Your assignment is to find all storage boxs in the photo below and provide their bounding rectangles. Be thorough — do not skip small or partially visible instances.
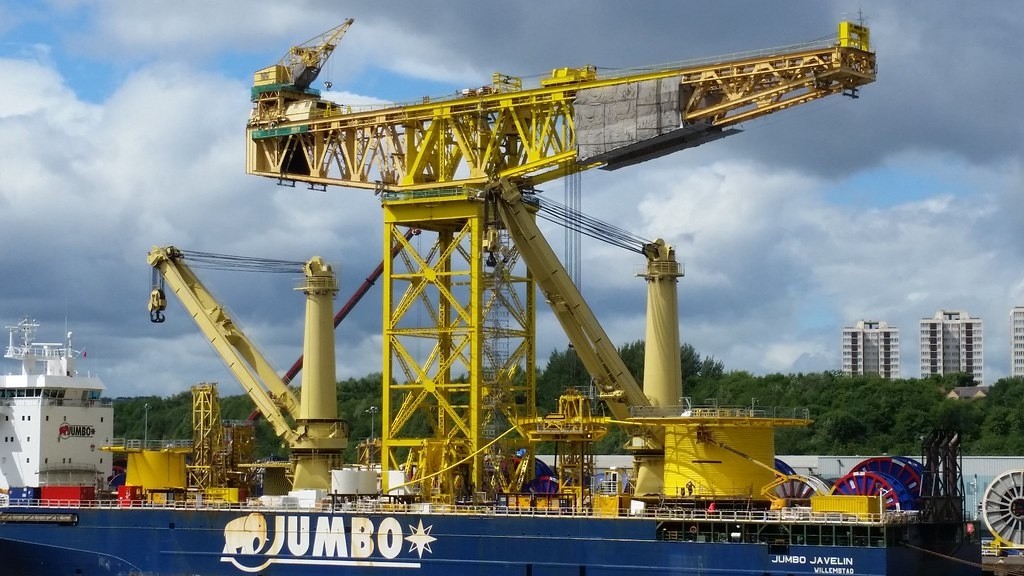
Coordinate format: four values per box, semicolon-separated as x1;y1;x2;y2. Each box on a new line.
40;486;94;508
811;495;886;521
498;495;569;515
9;487;40;506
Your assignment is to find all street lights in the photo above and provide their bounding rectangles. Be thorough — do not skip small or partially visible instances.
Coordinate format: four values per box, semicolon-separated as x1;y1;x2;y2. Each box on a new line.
144;401;152;453
364;405;378;465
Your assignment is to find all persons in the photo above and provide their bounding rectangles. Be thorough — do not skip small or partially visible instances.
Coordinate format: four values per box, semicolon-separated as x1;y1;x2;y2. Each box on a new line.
686;480;695;495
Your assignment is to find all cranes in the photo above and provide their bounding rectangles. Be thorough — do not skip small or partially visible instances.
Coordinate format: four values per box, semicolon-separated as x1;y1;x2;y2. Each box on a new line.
242;13;880;504
145;243;350;498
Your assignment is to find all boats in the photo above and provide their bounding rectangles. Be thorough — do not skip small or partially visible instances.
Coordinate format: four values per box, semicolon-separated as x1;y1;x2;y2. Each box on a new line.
0;14;985;576
